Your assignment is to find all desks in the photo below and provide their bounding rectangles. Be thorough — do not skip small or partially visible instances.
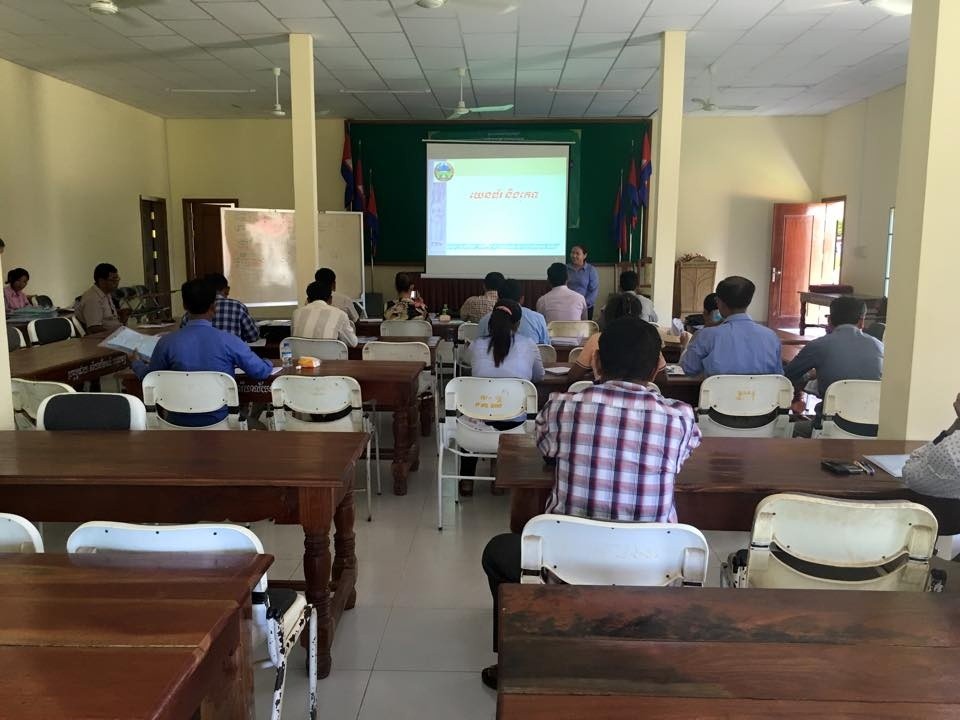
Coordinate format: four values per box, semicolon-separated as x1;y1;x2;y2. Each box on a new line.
114;359;426;496
252;337;440;431
0;431;370;676
770;329;816;364
0;551;274;720
530;362;703;407
496;584;960;719
555;336;682;363
9;318;183;392
0;285;960;720
254;317;463;376
493;434;928;532
5;307;74;347
797;291;888;335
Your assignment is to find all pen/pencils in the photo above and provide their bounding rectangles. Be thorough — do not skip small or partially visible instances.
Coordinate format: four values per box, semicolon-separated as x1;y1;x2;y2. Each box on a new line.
856;462;869;472
431;319;440;320
860;462;873;472
864;462;875;472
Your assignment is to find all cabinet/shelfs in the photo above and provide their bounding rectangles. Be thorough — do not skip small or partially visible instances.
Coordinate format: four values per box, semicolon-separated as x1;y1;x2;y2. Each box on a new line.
674;255;717;318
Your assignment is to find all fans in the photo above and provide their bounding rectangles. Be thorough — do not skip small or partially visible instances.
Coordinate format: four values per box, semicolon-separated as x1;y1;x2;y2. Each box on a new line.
432;68;514;120
820;0;912;18
687;63;759;112
377;0;518;15
69;0;166;27
262;67;288;117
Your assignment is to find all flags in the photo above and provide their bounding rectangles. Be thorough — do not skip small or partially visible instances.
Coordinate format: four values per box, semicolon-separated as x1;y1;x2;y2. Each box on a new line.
611;132;652;255
340;131;378;257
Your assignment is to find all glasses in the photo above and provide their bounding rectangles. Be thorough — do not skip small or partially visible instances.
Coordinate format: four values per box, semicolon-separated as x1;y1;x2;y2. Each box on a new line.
104;277;120;284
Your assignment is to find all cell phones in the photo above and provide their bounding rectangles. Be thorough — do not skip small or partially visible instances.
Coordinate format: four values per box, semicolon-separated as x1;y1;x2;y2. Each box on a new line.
840;463;864;474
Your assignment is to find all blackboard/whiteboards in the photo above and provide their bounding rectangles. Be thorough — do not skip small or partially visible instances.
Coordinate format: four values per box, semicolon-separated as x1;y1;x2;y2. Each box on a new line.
220;207;364;307
344;119;652;266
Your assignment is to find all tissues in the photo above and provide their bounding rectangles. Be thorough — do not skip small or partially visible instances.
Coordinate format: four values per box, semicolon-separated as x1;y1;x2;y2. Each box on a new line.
297;357;321;368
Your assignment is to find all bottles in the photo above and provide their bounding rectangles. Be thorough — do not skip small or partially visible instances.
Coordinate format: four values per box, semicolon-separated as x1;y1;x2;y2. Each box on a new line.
281;343;293;367
442;304;449;314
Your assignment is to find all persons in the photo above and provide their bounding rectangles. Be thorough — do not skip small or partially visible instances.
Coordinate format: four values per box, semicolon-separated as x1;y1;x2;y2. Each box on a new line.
479;279;551;346
180;273;259;342
459;299;544;496
481;315;702;689
783;297;884;439
679;275;784;429
76;263;124;334
565;244;599;321
290;281;358;348
305;268;360;328
567;293;668;388
620;271;658;321
127;279;272;427
536;262;588;323
460;271;505;324
902;393;960;594
383;272;432;324
3;268;32;311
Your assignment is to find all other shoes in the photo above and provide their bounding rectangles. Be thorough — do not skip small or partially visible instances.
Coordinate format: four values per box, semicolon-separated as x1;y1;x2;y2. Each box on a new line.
482;664;500;690
492;483;504;496
458;479;473;497
